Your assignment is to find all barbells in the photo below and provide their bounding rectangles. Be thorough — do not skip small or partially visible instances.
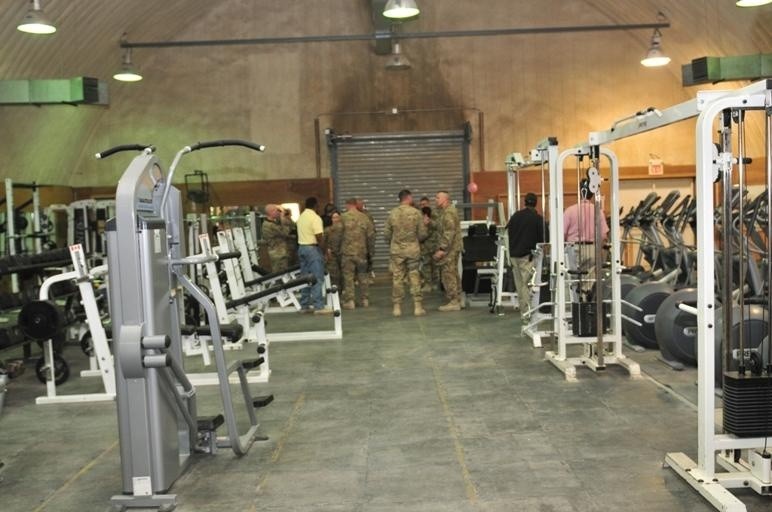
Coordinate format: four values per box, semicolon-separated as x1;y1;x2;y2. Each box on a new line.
18;290;104;342
35;330;96;386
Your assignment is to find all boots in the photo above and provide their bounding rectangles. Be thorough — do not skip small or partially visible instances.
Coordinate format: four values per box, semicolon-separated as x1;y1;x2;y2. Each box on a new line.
413;301;426;316
343;300;355;309
361;299;369;306
393;304;401;316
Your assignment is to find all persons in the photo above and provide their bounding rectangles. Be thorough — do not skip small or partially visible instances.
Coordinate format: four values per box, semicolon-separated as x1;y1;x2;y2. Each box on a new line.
258;197;377;314
384;189;426;317
432;191;461;312
562;178;609;304
504;192;550;324
420;197;439;293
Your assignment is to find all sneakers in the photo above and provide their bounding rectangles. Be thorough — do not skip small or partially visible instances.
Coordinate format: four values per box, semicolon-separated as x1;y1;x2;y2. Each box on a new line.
438;295;466;311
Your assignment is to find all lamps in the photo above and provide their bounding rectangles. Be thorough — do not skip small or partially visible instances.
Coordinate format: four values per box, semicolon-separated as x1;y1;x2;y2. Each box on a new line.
111;44;146;86
17;1;60;35
383;40;412;72
380;0;423;21
639;26;674;68
